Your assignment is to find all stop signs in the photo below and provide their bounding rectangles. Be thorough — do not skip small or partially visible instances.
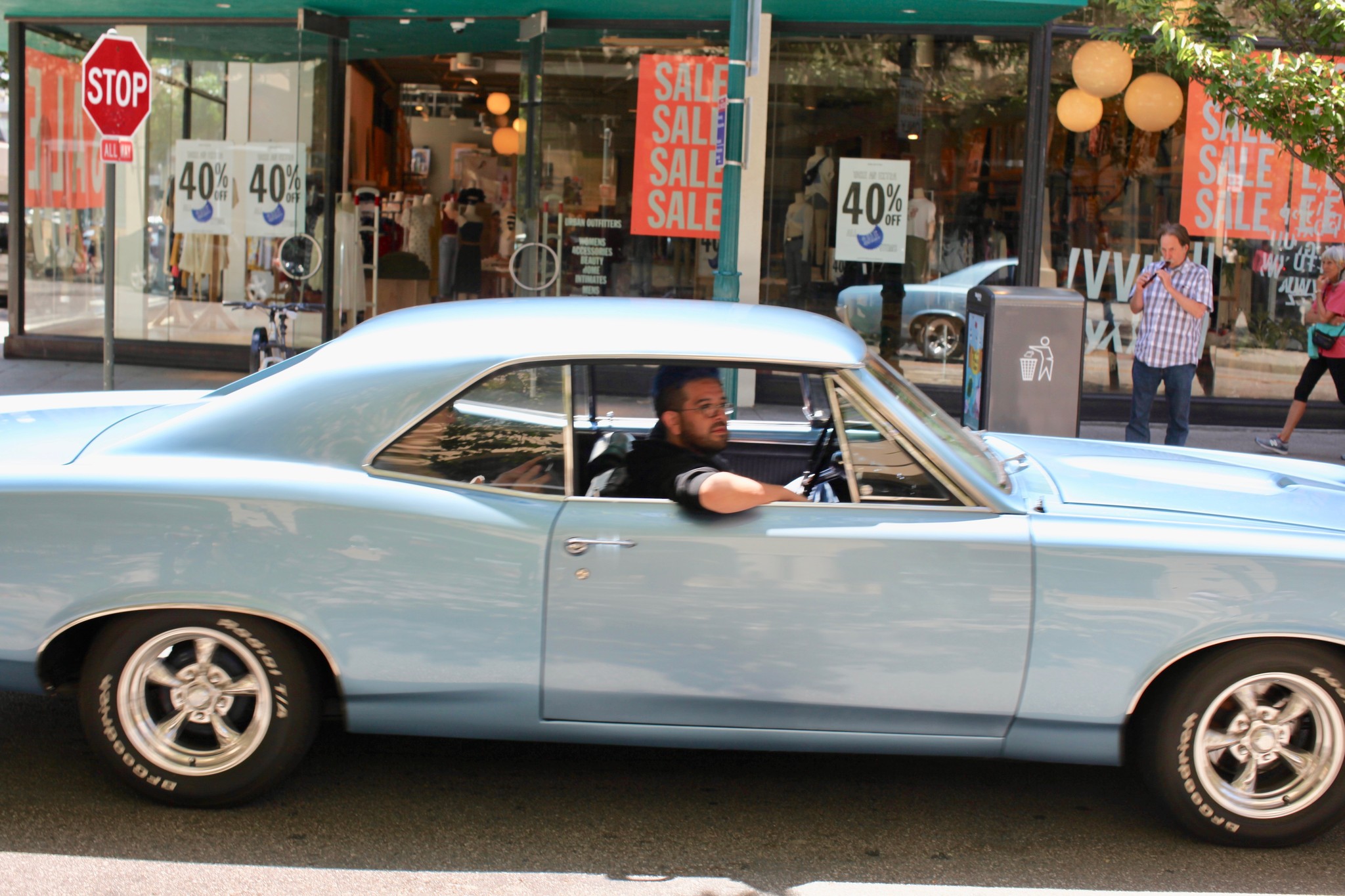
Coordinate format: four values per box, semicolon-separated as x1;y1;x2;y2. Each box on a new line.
80;36;152;137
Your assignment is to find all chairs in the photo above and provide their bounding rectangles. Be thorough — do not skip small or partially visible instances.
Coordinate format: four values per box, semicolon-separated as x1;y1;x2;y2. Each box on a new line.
590;428;639;501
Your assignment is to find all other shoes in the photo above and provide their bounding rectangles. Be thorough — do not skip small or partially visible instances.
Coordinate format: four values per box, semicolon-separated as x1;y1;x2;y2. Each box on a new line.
1255;434;1289;455
1341;454;1345;460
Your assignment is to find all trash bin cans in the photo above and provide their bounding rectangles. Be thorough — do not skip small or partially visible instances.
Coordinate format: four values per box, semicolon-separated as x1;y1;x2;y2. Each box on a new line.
960;283;1088;438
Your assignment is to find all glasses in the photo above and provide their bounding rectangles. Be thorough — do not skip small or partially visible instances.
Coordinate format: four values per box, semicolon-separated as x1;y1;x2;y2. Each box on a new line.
681;403;734;418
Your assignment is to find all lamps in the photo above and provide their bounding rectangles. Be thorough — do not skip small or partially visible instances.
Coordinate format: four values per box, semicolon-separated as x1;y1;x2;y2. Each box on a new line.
915;34;1183;134
415;53;527;157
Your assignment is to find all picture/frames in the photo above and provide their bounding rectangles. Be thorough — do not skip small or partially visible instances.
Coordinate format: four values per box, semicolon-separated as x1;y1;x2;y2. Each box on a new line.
450;143;478;180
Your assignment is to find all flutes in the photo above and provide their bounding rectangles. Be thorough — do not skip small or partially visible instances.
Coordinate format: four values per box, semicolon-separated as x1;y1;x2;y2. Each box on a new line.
1141;261;1171;289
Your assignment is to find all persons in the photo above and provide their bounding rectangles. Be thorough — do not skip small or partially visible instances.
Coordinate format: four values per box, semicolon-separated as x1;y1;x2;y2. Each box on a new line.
1126;222;1214;447
1251;240;1279;311
370;401;552;494
619;365;814;514
1257;247;1344;459
1222;239;1238;289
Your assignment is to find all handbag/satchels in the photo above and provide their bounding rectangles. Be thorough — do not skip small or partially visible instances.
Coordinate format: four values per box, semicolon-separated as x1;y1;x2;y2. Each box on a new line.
804;165;818;186
1312;329;1336;350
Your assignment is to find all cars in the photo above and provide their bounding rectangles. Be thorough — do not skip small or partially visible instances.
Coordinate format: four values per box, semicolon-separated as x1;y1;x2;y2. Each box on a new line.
835;256;1023;365
0;294;1344;851
